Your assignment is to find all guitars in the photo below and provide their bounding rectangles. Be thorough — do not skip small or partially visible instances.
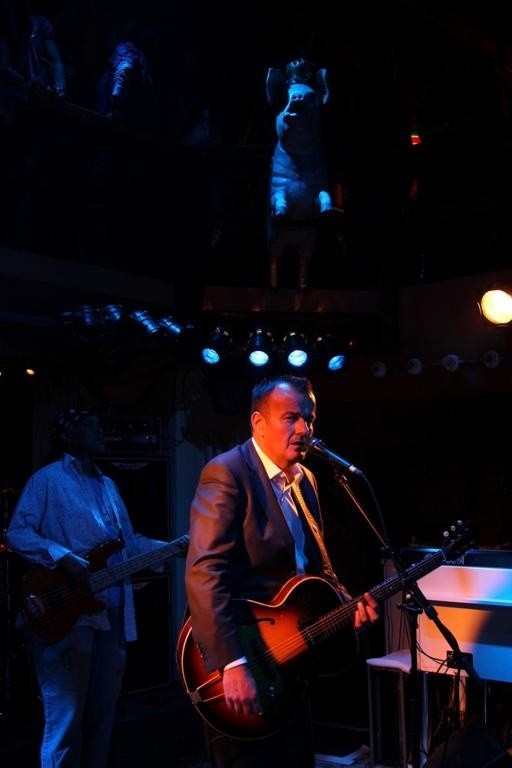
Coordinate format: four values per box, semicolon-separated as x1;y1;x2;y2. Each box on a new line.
175;519;477;741
2;535;189;652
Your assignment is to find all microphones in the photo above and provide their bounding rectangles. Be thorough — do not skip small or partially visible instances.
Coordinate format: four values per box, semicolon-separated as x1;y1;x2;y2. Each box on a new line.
305;437;363;477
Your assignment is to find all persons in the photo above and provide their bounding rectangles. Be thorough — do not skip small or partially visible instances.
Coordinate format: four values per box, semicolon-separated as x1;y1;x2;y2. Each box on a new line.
5;410;190;768
183;374;380;767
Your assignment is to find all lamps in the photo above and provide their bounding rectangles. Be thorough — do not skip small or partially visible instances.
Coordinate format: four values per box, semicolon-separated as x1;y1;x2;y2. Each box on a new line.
198;326;350;375
